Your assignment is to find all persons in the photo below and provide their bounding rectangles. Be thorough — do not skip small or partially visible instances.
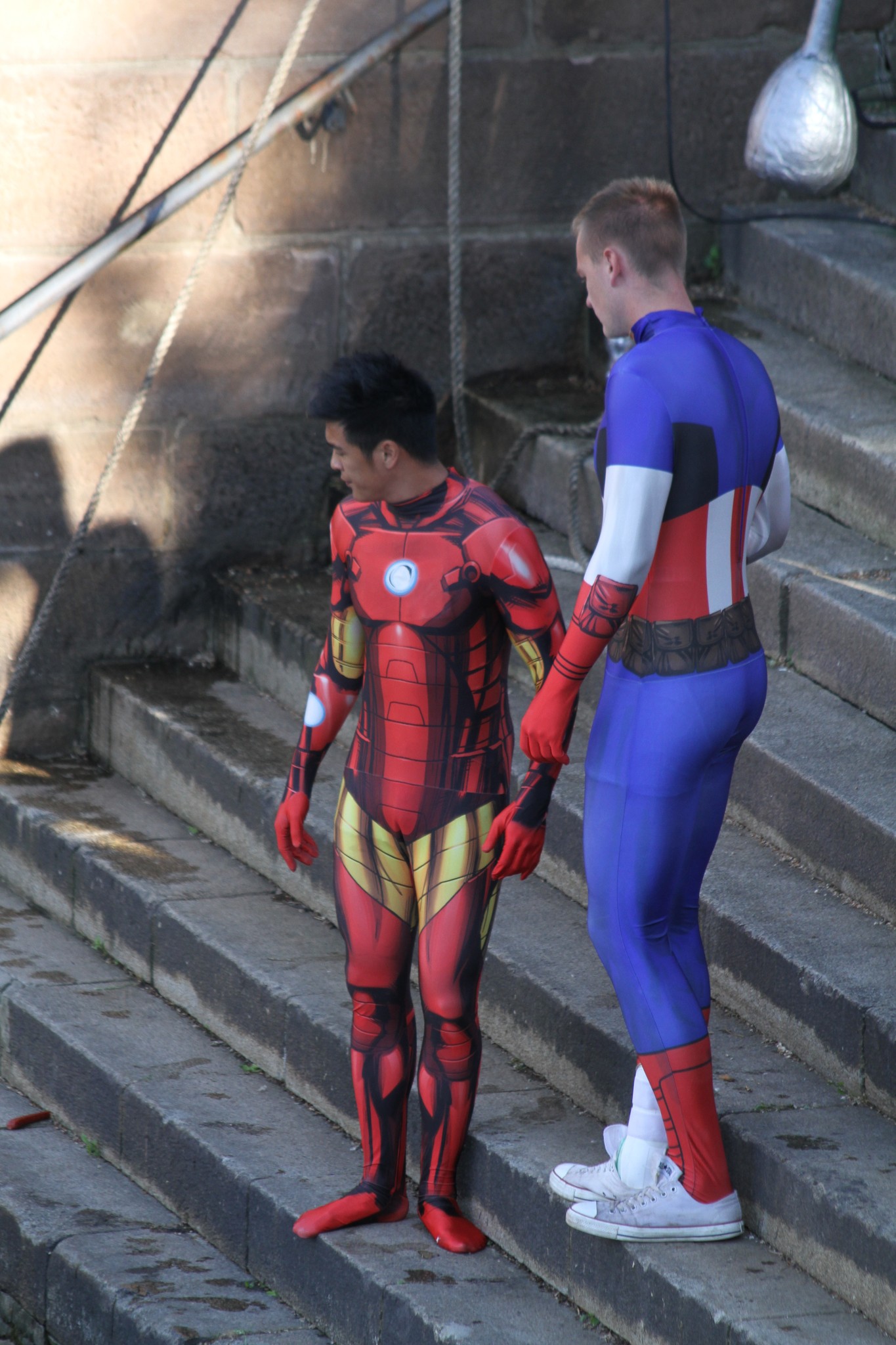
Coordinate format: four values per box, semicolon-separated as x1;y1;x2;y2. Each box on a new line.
518;178;794;1241
273;353;577;1259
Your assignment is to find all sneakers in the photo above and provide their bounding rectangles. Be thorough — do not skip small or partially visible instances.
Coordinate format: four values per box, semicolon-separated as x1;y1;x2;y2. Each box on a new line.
549;1123;646;1204
566;1154;745;1243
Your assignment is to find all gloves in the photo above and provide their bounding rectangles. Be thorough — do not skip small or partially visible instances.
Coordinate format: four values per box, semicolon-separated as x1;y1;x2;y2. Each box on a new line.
274;790;320;873
519;681;580;765
481;795;548;881
